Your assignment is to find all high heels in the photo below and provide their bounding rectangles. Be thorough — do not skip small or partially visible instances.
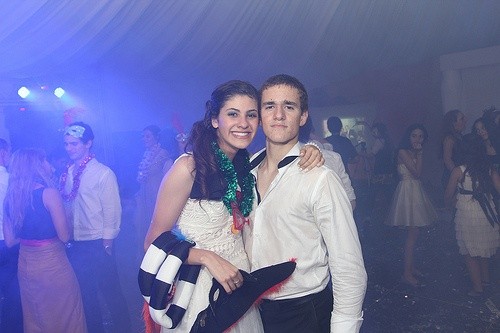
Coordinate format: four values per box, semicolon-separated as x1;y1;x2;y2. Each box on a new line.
400;266;426;287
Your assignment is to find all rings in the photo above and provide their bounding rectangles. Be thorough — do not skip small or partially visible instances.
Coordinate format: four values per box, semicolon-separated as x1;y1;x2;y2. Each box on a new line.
234;279;241;284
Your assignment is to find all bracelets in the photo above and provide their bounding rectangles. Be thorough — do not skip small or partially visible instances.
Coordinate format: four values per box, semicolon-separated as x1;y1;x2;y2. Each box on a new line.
103;244;112;249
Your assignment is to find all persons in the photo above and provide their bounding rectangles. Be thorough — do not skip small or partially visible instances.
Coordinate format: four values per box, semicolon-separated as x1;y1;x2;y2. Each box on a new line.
0;138;23;333
397;124;438;288
2;147;88;333
491;111;500;126
135;125;172;270
58;122;132;333
324;116;360;179
472;119;500;175
55;130;69;188
144;80;325;333
358;123;394;220
442;110;467;239
444;134;500;298
241;74;367;333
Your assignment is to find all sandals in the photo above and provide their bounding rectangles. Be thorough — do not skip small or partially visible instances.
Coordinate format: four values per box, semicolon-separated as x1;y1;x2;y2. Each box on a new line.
463;281;491;297
